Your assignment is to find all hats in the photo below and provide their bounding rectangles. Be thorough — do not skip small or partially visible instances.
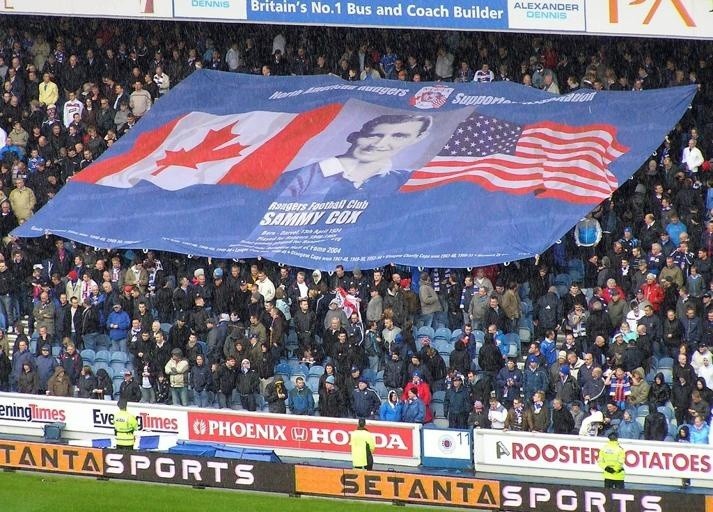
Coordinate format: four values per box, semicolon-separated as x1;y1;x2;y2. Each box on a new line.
0;24;713;440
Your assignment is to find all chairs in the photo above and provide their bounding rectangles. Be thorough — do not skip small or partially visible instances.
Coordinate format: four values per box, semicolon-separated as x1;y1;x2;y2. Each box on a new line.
29;257;672;445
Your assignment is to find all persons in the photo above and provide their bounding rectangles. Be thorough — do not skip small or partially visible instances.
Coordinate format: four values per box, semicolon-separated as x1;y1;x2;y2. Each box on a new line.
276;115;433;197
1;74;161;399
552;84;712;274
597;432;625;488
114;399;137;450
0;15;712;89
145;253;712;446
351;418;374;470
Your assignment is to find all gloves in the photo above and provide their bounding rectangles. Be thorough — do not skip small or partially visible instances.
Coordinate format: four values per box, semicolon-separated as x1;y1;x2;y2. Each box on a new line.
605;467;615;473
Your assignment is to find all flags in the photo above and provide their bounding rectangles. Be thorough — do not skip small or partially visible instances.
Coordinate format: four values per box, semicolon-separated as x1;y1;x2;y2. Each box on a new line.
72;103;342;191
399;110;629;203
